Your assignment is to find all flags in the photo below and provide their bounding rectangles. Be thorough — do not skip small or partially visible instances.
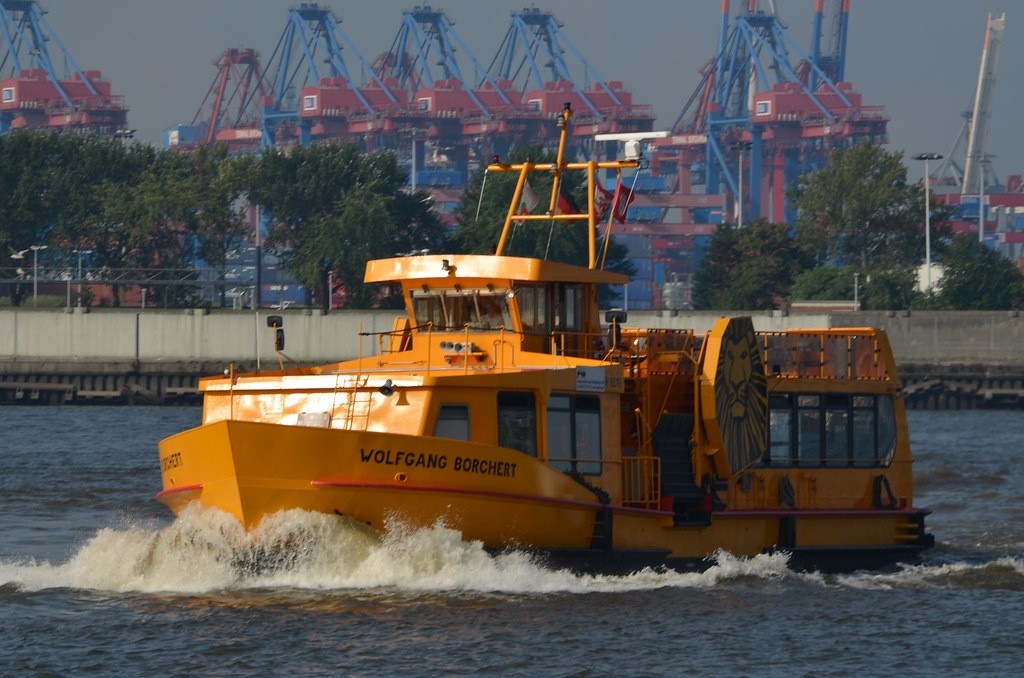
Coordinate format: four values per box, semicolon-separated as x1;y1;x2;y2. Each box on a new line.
515;179;539;227
594;180;613;226
556;182;583;225
613;184;634;224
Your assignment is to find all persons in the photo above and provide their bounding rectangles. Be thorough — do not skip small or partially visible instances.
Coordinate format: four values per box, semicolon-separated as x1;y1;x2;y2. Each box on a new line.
10;274;23;307
479;303;505;329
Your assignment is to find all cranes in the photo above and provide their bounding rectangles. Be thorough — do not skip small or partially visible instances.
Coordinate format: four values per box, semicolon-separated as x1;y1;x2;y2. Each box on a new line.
0;1;893;311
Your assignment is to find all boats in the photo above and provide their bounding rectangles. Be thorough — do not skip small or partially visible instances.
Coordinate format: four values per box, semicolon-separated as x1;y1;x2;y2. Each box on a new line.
154;100;934;580
